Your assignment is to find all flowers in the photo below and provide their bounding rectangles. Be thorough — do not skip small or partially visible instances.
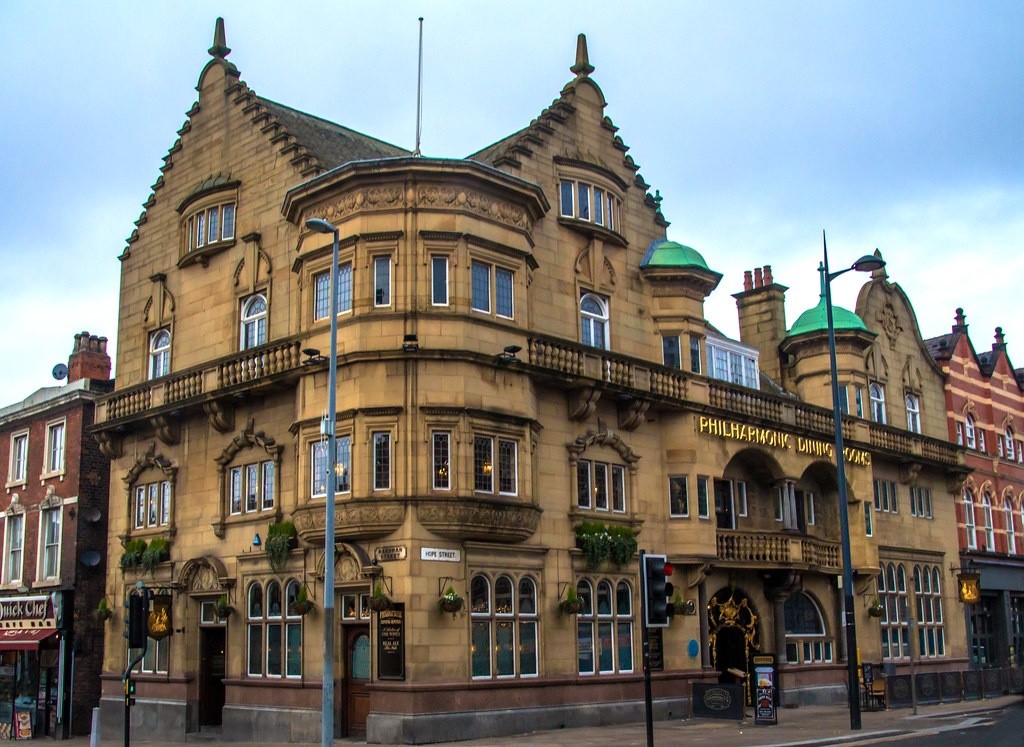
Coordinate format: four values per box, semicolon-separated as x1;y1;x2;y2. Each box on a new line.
872;600;883;611
438;586;468;620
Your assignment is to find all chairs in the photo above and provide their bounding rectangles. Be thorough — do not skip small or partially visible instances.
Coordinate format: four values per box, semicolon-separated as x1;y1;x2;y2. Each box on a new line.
844;675;886;711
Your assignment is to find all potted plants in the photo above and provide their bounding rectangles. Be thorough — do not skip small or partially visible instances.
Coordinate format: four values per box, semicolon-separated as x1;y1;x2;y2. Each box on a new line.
558;580;585;615
672;589;689;615
368;579;390;612
90;598;111;618
290;584;315;615
212;595;236;617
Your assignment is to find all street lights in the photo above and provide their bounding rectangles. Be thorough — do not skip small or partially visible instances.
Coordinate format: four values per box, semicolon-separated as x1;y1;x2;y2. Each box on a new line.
303;217;336;745
817;228;887;730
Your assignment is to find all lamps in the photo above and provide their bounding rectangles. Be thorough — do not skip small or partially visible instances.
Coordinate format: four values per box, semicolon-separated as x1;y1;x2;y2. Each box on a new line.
303;349;320;356
503;345;522;353
403;334;419;342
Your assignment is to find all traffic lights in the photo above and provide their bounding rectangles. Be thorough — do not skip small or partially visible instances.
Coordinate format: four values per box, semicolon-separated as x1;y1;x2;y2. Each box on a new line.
640;553;674;630
123;592;142;650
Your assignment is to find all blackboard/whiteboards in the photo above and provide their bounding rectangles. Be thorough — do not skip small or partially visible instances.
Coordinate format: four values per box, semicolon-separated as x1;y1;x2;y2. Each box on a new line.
755;687;774;719
